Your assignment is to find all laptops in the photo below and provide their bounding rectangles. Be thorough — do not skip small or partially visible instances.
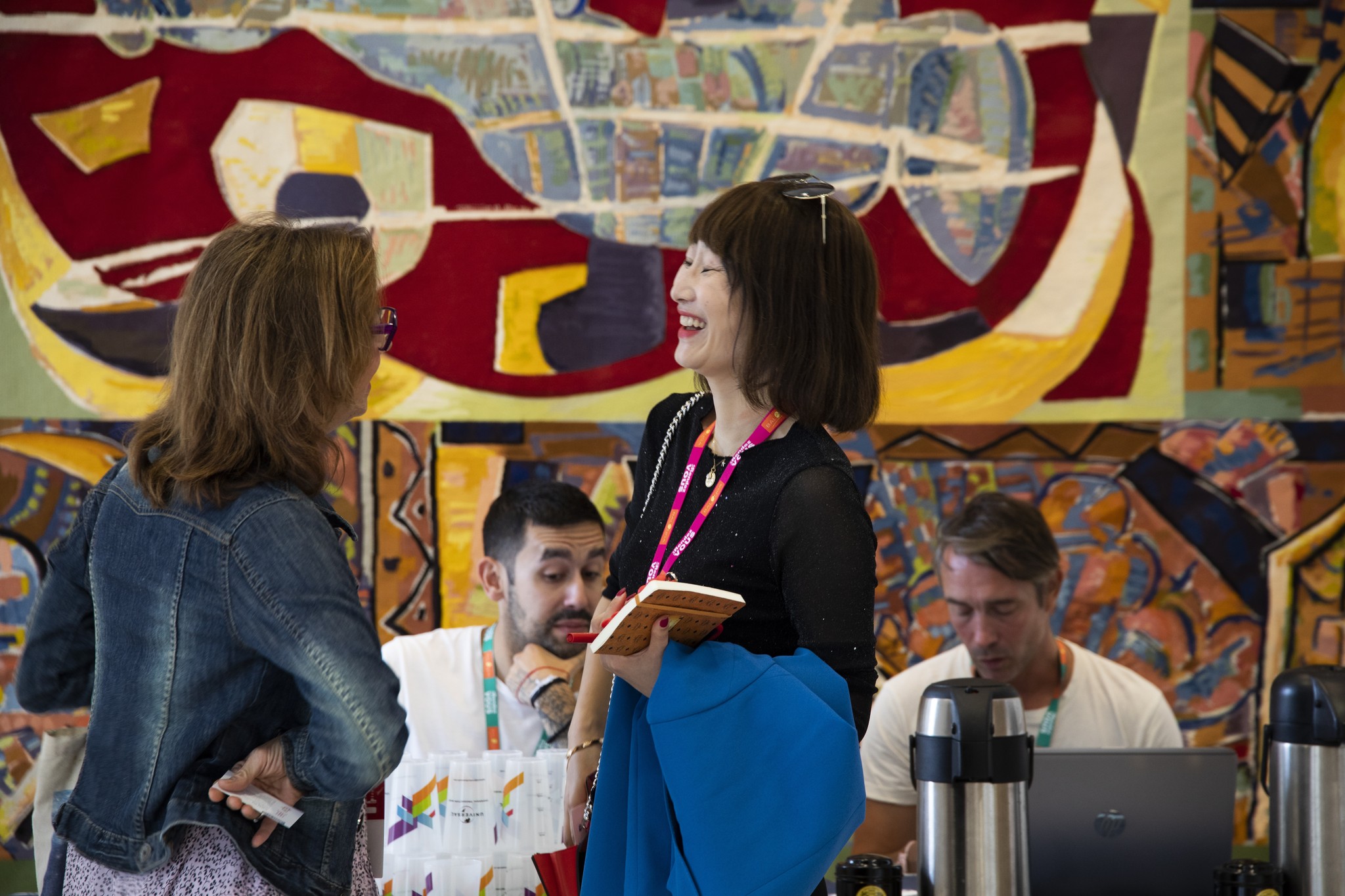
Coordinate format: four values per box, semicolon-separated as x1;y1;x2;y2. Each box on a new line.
1027;747;1237;896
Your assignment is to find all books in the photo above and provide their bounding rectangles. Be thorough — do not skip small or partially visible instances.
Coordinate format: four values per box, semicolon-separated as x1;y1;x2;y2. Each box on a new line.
589;580;746;655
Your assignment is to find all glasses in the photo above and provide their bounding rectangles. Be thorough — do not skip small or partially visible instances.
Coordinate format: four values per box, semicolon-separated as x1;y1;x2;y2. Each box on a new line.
370;306;398;351
761;172;836;252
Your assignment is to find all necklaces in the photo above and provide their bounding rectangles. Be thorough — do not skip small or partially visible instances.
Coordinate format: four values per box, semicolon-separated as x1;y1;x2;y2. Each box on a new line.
705;432;742;488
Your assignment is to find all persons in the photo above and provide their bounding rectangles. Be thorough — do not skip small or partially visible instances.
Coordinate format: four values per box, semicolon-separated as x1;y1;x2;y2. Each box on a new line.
561;172;882;896
379;482;607;890
19;213;410;896
853;486;1184;872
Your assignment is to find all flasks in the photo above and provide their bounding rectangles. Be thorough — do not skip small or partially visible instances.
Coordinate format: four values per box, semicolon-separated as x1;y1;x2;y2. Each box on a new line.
1261;665;1344;896
835;852;903;896
908;677;1035;896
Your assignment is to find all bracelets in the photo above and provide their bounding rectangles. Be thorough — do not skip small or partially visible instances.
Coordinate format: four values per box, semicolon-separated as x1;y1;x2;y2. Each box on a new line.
547;719;572;744
517;666;570;706
531;677;568;708
567;738;604;757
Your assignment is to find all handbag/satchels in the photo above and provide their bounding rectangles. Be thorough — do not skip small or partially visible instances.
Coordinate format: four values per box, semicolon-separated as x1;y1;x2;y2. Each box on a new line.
531;769;600;896
32;725;89;896
